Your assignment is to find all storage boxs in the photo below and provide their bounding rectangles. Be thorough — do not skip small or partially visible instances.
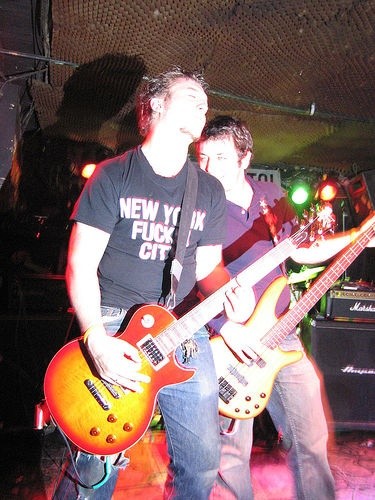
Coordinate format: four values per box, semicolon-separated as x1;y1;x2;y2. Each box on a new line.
318;288;375;323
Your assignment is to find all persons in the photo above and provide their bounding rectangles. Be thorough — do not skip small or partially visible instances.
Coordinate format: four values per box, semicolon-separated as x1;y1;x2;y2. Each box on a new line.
189;110;375;500
48;65;256;500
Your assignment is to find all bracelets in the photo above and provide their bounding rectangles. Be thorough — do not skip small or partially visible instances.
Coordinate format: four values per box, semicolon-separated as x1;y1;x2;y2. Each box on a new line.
81;325;96;345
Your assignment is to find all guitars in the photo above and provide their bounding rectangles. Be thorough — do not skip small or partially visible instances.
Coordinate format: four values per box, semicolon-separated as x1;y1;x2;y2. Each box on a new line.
207;218;375;420
42;204;341;456
